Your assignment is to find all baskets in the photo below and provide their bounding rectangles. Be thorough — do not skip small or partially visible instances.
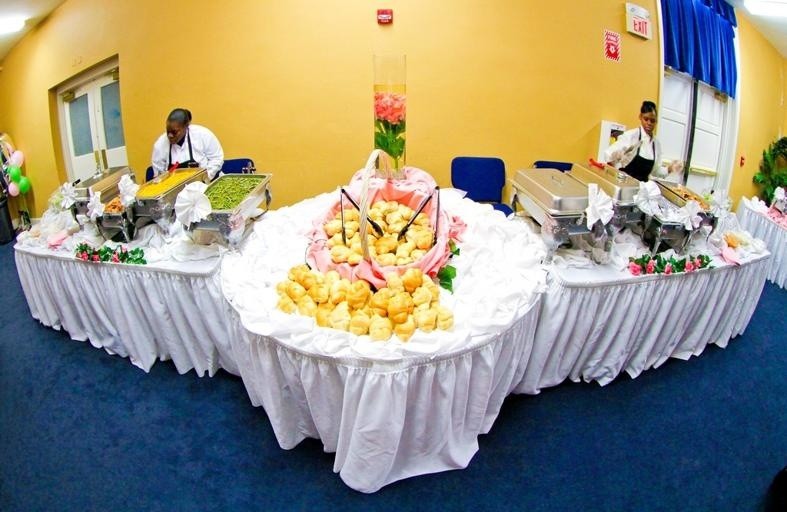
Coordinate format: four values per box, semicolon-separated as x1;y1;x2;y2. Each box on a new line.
307;149;452;289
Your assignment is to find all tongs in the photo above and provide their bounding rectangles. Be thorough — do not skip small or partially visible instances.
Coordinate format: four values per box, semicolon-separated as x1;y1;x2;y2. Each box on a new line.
397;183;440;245
588;158;626;180
153;163;179;183
337;185;383;246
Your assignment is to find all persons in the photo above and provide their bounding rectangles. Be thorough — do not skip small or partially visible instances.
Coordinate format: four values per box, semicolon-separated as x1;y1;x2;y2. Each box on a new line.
604;101;682;182
152;109;224;184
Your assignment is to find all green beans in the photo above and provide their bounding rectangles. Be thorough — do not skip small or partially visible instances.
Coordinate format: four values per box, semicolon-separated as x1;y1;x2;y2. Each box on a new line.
206;177;262;209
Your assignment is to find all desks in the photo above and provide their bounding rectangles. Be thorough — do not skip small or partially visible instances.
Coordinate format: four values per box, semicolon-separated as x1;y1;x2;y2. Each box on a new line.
518;197;773;394
13;206;265;378
734;197;786;292
220;187;549;492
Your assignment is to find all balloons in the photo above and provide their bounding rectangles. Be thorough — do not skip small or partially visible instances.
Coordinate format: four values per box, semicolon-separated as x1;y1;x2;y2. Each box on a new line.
7;150;30;197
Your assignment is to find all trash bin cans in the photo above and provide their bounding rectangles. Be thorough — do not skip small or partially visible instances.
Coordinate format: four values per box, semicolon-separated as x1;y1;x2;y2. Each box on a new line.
0;196;15;246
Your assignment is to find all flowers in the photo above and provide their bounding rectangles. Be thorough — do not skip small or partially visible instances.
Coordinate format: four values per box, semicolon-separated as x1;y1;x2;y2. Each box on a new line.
619;253;712;276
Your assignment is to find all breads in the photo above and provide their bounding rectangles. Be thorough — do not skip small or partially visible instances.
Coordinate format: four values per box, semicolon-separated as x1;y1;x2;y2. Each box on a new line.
278;199;452;343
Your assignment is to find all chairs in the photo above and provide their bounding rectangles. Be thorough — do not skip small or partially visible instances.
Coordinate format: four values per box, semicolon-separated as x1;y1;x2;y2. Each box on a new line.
450;157;514;217
